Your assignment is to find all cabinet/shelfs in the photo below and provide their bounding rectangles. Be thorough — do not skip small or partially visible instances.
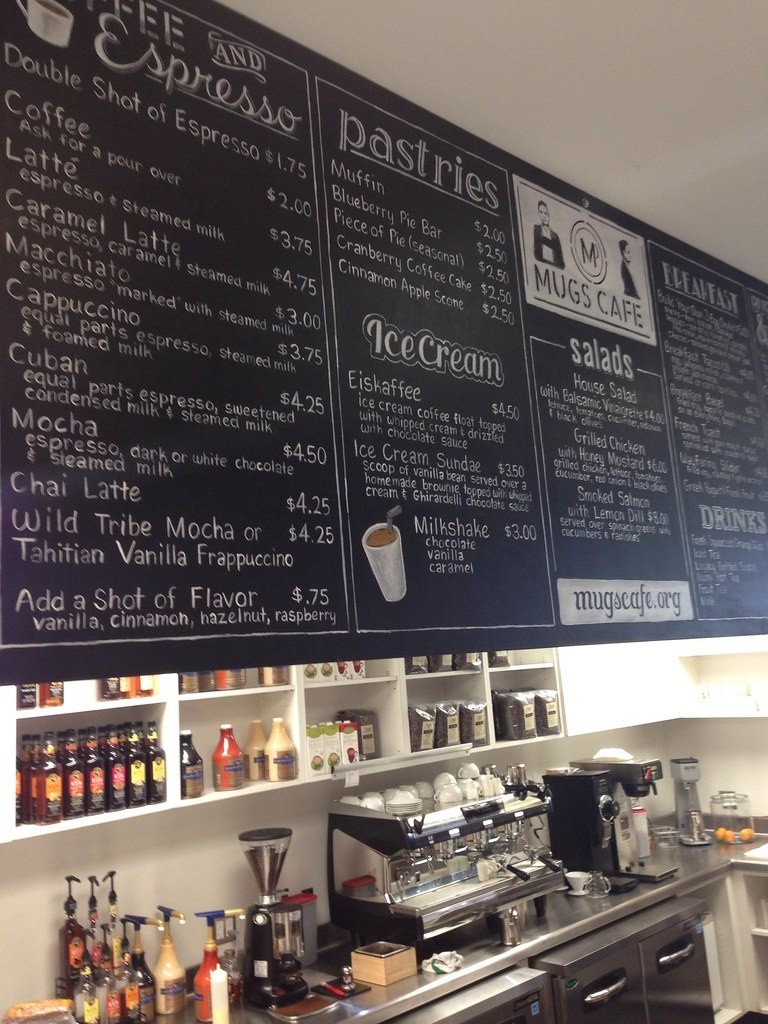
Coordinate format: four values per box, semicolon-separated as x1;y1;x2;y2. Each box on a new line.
0;652;567;845
676;869;768;1024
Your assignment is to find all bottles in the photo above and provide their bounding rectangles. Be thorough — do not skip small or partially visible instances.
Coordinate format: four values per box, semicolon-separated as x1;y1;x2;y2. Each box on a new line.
152;906;187;1014
58;876;87;1000
223;949;242;1010
103;871;126;975
96;923;121;1024
193;909;244;1023
82;876;103;972
16;666;298;829
73;929;100;1024
125;914;163;1024
117;919;140;1023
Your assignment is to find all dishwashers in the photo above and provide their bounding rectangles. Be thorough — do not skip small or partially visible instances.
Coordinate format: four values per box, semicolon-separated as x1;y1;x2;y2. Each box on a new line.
531;896;714;1024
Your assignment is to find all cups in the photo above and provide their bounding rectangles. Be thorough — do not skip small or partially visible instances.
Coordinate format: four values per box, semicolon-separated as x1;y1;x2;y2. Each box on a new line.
477;862;493;881
483;860;500;878
565;872;592;895
586;870;611;899
711;790;755;844
342;763;506;814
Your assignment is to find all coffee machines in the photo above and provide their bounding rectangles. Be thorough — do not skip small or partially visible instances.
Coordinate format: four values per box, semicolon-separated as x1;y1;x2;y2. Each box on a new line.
669;758;712;846
325;768;568;970
569;756;677;882
542;767;639;894
237;827;338;1020
